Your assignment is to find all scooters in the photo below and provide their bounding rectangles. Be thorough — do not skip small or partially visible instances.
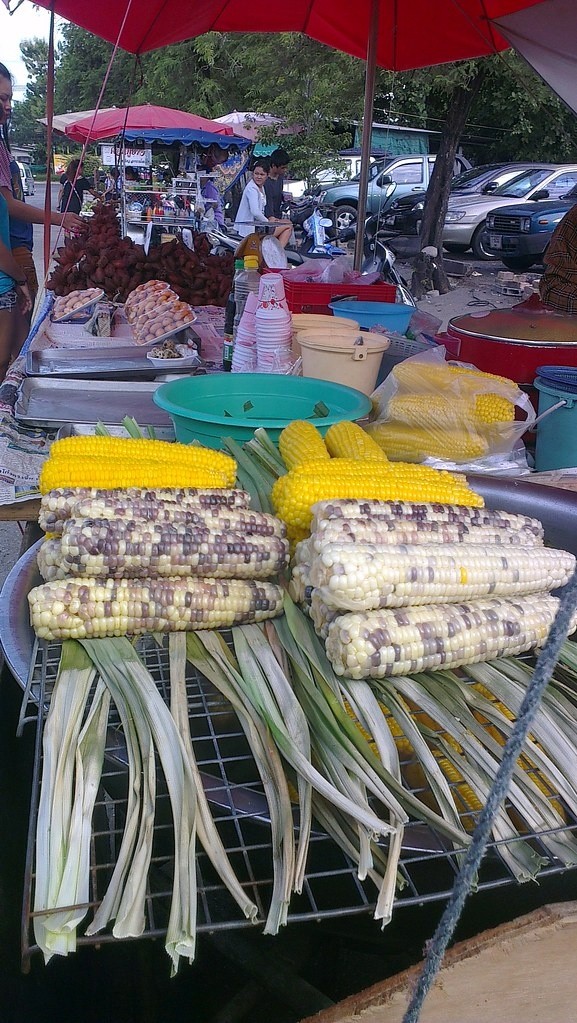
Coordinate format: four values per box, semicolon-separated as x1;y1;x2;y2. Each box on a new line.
190;181;417;307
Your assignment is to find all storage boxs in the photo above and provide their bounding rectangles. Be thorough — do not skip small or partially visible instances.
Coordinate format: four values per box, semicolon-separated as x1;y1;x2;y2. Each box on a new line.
261;267;397;316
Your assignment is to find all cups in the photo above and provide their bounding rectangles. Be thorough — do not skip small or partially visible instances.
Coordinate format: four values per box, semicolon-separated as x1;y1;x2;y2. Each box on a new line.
232;273;292;374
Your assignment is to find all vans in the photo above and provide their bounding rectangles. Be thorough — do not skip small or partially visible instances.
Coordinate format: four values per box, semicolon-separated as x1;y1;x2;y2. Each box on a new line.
15;160;36;196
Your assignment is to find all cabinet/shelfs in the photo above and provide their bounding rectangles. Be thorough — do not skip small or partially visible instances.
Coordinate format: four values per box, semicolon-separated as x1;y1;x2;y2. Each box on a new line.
123;173;218;227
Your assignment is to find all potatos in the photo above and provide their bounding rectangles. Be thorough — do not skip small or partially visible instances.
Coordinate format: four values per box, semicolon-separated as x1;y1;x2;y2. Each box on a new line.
44;202;230;307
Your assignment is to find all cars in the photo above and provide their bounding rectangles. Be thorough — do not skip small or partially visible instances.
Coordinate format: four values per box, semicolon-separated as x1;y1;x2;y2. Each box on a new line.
381;161;577;251
78;171;108;190
482;184;577;270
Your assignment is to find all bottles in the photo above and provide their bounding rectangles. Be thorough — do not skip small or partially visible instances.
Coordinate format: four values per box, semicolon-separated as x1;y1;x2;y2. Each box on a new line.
141;201;195;223
222;259;245;373
233;256;262;348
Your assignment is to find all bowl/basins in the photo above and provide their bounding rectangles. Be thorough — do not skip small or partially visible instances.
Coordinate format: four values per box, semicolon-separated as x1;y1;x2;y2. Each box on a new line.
147;349;198;368
327;300;418;337
152;372;373;454
0;471;577;858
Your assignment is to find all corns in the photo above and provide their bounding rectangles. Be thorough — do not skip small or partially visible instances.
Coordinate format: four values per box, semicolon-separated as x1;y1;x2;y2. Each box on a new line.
363;360;520;461
287;498;577;680
270;419;483;551
27;435;292;639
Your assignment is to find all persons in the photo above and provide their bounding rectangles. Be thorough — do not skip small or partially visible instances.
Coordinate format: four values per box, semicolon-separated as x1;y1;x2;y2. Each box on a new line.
58;160;103;239
263;150;289;223
0;62;183;388
540;204;577;317
202;168;228;233
234;161;292;249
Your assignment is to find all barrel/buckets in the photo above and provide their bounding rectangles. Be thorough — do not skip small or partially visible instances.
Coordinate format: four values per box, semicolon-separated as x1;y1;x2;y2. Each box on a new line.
286;314;390;397
527;377;577;473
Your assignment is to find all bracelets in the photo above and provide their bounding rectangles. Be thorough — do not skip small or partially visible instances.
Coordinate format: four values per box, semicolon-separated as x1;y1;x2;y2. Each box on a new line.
17;275;28;287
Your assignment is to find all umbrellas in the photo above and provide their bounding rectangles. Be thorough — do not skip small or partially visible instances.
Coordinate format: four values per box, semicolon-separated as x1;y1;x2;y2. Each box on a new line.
29;0;576;274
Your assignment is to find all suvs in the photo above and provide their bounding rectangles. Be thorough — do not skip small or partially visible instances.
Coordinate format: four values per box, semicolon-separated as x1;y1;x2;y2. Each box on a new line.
282;156;377;197
312;156;478;240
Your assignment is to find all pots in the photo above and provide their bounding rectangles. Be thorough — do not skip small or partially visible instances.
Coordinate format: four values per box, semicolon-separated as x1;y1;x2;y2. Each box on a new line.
422;293;577;447
